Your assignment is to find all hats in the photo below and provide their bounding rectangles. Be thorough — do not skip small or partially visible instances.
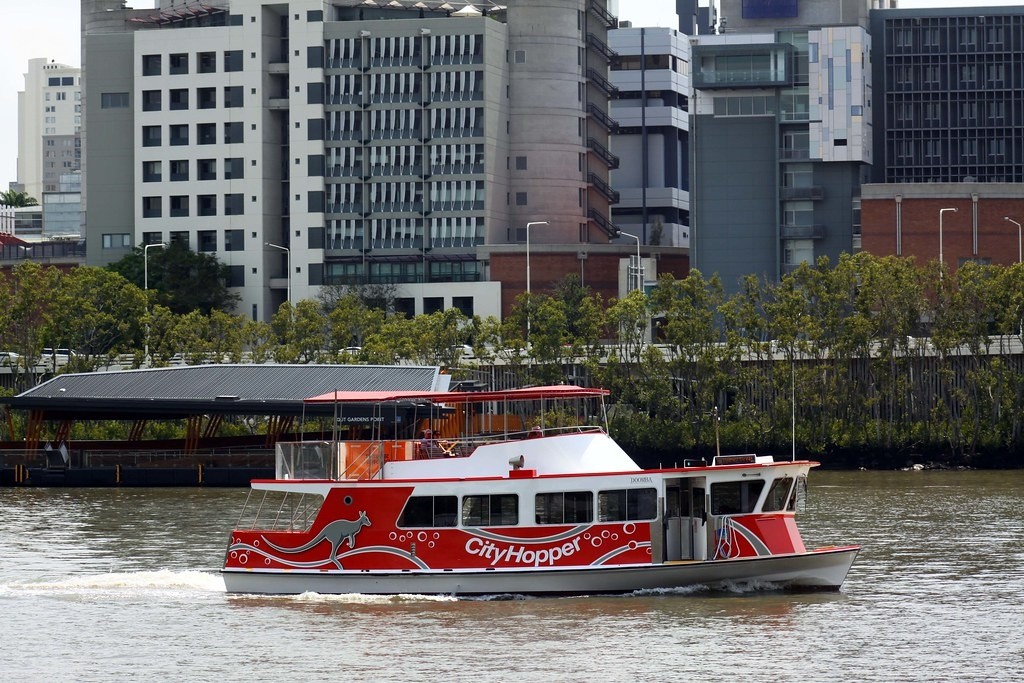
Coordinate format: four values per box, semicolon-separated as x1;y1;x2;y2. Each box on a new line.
424;429;432;433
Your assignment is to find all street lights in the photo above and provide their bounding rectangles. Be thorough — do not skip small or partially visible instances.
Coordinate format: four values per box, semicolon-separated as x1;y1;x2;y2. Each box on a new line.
144;244;166;364
939;207;958;289
264;241;291;319
527;222;550;358
1004;217;1022;267
617;230;642;341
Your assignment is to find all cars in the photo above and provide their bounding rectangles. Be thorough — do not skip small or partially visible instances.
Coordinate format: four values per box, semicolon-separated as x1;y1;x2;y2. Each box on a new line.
0;348;30;363
338;344;472;355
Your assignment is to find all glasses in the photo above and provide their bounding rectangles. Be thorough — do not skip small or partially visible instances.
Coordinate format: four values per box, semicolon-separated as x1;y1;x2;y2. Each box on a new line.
435;434;441;437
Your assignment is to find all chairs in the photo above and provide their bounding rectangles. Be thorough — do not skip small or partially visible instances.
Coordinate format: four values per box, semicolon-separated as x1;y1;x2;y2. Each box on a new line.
425;446;474;458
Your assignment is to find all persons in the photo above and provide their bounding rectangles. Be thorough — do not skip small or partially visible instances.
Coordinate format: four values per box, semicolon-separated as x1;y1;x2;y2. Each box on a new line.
432;430;446;452
418;430;425;439
421;429;437;450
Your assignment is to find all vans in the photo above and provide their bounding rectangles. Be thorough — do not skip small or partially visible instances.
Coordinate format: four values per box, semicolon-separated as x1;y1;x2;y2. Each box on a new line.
35;348;86;367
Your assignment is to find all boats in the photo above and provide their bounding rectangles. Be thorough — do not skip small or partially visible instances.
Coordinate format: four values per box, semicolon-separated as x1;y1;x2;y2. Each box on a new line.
222;383;860;591
